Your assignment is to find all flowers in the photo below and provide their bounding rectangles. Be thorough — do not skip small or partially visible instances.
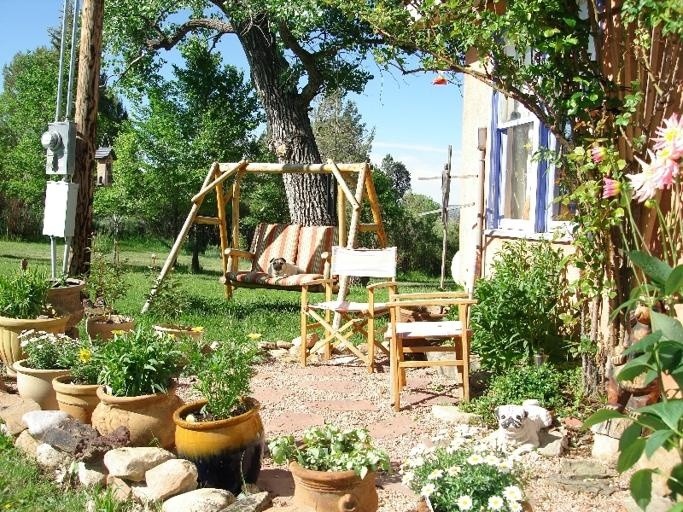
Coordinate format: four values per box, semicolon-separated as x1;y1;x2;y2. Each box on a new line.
403;440;531;512
270;420;392;478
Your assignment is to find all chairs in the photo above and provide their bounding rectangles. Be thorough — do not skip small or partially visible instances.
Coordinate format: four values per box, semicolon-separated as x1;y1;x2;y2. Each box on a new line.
296;244;399;374
384;238;481;411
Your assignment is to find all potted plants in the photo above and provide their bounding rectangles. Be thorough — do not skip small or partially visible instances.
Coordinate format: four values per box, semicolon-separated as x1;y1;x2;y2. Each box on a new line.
170;334;264;490
1;260;188;449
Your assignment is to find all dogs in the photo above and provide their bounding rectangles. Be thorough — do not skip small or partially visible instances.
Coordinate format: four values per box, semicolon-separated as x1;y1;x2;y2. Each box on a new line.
493;399;553;455
268;257;303;284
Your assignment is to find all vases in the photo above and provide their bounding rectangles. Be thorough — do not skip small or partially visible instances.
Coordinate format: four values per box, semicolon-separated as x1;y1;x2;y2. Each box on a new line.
286;456;380;512
